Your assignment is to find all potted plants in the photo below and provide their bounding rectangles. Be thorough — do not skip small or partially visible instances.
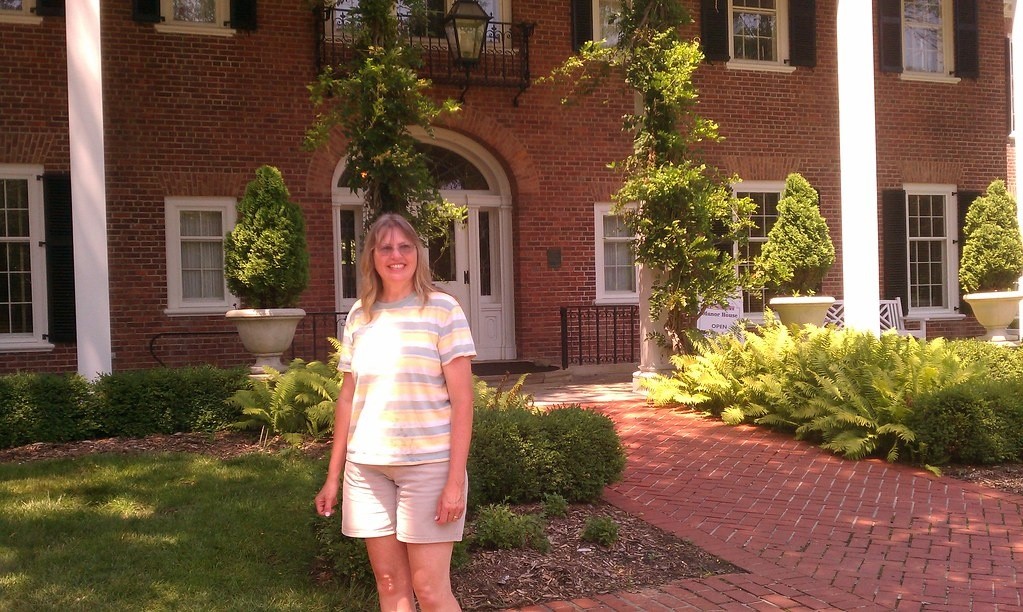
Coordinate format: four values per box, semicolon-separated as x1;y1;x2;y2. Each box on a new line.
225;165;309;376
755;171;837;334
960;178;1023;347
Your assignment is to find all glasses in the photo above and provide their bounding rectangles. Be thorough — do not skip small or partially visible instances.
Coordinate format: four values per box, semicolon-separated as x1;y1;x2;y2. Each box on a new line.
372;242;417;255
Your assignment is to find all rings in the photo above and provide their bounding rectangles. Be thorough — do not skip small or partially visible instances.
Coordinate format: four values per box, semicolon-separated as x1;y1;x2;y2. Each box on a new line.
454;516;458;519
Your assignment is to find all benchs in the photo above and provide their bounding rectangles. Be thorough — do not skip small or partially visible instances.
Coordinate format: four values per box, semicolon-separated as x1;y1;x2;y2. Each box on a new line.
821;297;929;345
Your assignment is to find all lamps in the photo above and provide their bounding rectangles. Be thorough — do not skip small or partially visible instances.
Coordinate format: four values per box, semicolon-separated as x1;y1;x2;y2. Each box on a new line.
442;0;493;103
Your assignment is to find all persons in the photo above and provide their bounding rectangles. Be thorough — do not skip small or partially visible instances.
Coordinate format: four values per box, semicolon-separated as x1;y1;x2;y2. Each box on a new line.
316;214;478;612
